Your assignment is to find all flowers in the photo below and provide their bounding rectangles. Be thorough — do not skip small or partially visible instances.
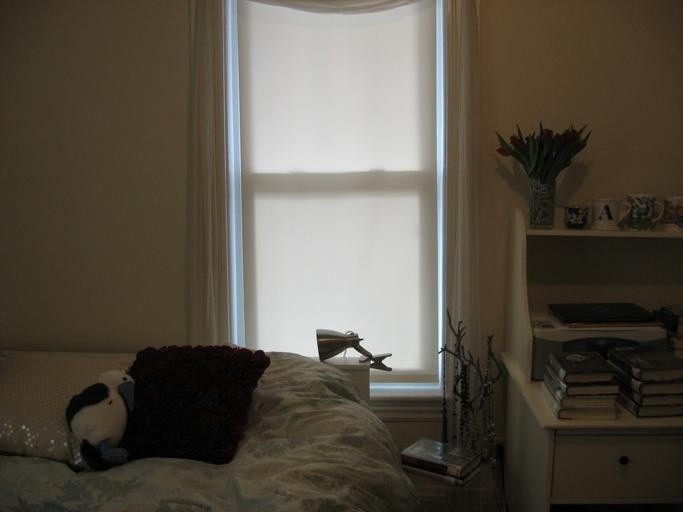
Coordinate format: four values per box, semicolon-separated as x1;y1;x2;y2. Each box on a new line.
496;122;591;184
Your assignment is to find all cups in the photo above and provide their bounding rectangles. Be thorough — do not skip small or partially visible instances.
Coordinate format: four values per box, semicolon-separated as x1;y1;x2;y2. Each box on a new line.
563;204;589;229
589;197;632;231
628;194;665;231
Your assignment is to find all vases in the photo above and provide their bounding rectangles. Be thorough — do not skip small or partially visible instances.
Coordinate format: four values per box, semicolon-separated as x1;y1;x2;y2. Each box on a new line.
530;179;555;229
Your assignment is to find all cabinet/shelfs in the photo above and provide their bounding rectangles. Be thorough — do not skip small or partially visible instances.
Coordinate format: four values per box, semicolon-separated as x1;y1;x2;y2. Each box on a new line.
501;206;682;511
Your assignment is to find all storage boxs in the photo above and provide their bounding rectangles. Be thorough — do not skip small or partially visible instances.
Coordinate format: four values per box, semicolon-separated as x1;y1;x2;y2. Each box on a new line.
531;318;666;380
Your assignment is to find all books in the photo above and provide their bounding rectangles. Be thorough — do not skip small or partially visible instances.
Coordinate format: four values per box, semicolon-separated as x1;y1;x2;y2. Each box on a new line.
401;463;485;488
401;437;488;479
539;302;683;421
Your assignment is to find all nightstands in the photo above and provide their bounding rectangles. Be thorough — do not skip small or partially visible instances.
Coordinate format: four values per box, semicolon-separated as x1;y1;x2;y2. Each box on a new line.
418;471;500;511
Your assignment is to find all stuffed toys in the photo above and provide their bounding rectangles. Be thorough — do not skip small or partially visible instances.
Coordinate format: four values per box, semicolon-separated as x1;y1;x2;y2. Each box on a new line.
66;369;135;474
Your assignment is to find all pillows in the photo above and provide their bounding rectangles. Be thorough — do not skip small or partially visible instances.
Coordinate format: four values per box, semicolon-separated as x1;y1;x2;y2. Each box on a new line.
119;345;271;464
0;351;136;470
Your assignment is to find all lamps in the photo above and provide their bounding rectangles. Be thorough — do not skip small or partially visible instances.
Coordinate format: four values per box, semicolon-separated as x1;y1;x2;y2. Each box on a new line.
316;329;392;370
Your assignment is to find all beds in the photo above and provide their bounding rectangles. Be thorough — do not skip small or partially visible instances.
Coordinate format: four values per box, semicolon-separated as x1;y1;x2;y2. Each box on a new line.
2;352;417;511
